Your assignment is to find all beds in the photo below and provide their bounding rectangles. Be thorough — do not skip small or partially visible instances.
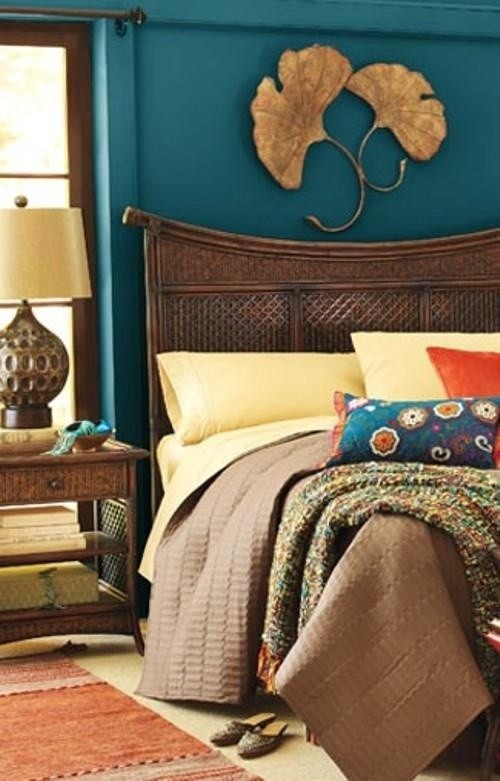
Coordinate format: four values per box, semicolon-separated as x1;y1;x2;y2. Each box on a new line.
122;207;500;781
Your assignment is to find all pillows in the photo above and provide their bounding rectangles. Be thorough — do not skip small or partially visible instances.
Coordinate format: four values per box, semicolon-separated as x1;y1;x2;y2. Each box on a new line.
319;390;500;468
154;349;368;448
425;345;500;400
350;332;500;402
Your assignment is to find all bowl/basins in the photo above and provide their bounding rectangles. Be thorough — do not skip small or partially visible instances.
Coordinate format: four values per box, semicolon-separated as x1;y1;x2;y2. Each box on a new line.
54;429;116;454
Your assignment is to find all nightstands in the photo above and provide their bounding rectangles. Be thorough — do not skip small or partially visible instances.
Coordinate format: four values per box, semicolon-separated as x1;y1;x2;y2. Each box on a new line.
0;439;155;658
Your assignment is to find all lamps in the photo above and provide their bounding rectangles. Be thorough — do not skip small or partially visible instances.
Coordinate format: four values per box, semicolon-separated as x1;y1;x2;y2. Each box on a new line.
0;196;93;427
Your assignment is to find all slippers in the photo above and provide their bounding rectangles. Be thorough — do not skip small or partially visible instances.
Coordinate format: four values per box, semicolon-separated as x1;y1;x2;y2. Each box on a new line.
208;711;277;747
237;719;289;758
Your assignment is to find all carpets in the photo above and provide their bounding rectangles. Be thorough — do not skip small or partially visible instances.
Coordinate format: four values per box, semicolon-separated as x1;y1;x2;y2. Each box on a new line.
2;649;262;781
